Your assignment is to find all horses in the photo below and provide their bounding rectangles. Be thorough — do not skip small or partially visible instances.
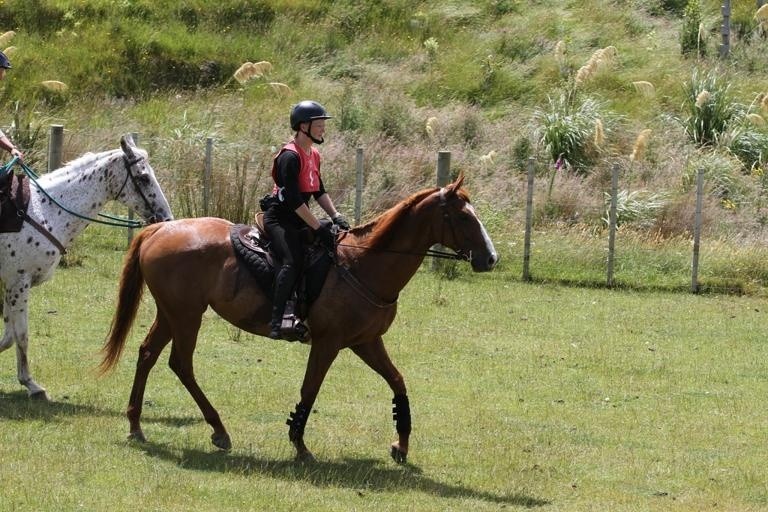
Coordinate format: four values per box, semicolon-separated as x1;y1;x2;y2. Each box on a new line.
88;170;500;467
0;133;174;403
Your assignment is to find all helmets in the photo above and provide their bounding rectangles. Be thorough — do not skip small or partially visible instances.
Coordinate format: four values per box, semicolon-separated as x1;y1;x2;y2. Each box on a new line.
291;99;333;133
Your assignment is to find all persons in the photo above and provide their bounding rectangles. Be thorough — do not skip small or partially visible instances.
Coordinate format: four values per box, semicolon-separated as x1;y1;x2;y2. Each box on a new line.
260;99;350;340
0;52;24;164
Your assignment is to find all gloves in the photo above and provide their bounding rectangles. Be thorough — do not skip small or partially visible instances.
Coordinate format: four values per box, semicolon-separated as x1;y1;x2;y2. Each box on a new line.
332;212;350;230
314;223;332;242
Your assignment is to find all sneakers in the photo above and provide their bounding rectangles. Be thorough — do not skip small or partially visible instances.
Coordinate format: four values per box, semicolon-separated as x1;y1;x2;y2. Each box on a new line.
270;322;310;342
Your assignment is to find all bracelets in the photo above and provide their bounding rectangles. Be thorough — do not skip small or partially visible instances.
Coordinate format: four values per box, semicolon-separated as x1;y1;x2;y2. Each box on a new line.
9;146;17;151
